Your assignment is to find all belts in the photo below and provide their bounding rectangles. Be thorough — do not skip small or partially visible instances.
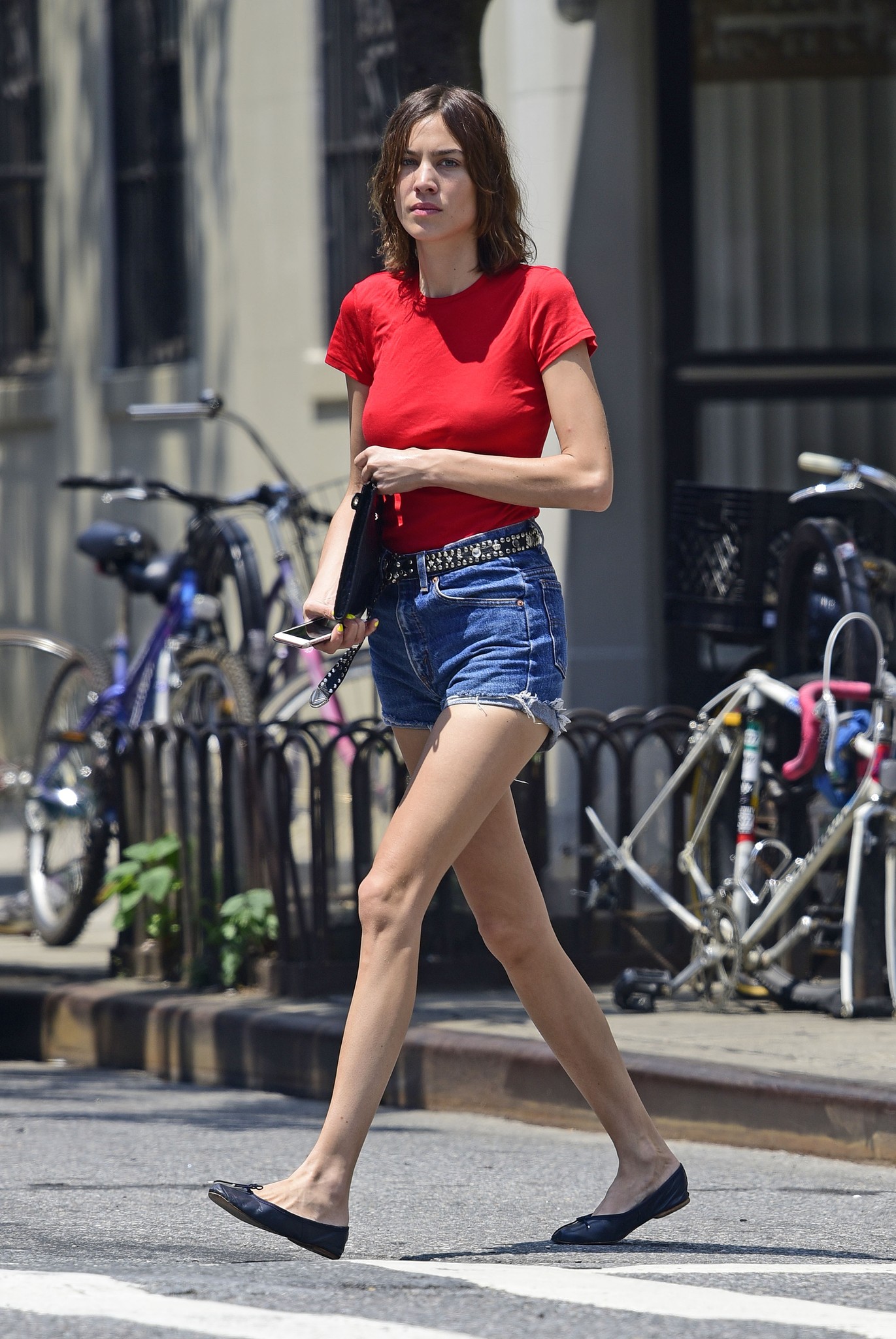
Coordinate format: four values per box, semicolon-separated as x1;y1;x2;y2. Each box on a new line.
311;526;544;711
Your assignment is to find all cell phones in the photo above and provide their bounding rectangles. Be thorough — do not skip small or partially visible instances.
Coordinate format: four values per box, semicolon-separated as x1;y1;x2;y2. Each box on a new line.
272;617;346;650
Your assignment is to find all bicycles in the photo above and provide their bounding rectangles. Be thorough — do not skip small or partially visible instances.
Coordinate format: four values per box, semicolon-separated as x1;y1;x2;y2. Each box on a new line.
19;389;411;946
767;450;895;1003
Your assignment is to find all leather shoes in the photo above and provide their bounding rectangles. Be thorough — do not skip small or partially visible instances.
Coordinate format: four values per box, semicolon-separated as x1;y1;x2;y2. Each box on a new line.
208;1180;349;1259
551;1163;690;1246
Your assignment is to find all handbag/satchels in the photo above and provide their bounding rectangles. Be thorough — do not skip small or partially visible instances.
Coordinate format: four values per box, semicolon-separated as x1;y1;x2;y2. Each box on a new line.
302;483;366;621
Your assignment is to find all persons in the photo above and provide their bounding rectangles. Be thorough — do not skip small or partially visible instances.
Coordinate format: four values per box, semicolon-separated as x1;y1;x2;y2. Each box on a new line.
207;85;691;1265
